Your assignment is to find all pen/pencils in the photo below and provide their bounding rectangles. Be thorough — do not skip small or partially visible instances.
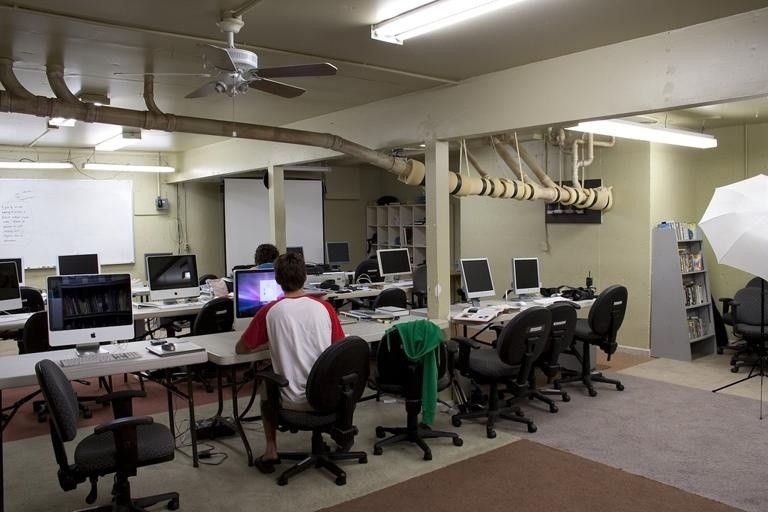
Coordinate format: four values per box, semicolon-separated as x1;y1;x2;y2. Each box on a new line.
377;319;391;323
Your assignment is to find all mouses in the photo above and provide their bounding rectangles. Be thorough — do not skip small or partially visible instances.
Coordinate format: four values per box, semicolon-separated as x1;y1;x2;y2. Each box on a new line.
516;301;526;306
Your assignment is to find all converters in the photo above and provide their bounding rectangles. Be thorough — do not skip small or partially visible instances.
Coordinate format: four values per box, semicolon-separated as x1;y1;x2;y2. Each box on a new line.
197;449;211;459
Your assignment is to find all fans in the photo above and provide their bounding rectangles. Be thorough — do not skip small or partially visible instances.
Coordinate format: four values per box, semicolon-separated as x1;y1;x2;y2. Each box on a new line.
107;4;338;136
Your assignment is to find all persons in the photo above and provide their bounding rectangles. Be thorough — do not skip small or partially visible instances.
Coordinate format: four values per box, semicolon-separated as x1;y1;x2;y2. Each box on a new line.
251;244;280;268
234;255;344;464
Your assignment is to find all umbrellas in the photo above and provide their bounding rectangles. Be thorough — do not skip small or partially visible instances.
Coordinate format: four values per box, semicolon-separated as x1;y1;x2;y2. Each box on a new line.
698;173;767;374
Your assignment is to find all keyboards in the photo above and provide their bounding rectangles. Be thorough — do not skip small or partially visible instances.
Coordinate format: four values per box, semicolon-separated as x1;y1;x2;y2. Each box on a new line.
393;281;413;287
533;297;571;304
59;352;141;367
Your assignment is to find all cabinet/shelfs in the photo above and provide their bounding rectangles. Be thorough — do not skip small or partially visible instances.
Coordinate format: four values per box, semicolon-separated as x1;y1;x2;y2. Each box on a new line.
363;201;456;272
650;222;720;365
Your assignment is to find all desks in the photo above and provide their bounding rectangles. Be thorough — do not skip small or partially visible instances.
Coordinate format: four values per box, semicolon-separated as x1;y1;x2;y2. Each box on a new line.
3;302;160;354
332;278;425;311
407;286;600;382
136;282;328;338
184;313;410;469
0;341;207;473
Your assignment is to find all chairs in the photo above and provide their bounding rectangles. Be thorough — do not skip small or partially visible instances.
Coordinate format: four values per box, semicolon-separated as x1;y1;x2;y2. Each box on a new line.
344;287;407;310
238;333;368;487
3;311;111;430
365;319;462;462
199;274;218;286
355;253;385;285
3;286;91;387
159;297;254;393
554;282;628;400
487;297;578;416
719;274;767;374
25;356;180;512
448;304;539;437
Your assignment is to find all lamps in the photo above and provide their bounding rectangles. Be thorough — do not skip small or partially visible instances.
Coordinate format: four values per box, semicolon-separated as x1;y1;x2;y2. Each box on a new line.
45;91;110;130
561;115;718;154
367;0;522;47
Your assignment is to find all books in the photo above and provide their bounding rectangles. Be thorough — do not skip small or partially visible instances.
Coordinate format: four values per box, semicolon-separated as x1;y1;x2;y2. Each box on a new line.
661;219;706;340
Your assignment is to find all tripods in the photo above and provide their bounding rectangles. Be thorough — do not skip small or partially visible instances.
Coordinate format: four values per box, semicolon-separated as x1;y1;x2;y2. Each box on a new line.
712;279;768;419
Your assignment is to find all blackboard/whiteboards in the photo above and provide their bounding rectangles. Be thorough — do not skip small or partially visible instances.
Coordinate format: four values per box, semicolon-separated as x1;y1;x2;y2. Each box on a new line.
0;179;136;269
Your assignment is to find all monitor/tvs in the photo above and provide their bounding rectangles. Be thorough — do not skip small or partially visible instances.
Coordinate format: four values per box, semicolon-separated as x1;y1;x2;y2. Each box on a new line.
511;257;541;302
0;253;200;358
459;258;496;309
326;243;350;271
233;268;285;331
376;248;413;283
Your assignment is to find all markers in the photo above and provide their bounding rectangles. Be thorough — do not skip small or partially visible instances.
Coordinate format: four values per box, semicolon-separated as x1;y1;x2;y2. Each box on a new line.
28;265;56;270
